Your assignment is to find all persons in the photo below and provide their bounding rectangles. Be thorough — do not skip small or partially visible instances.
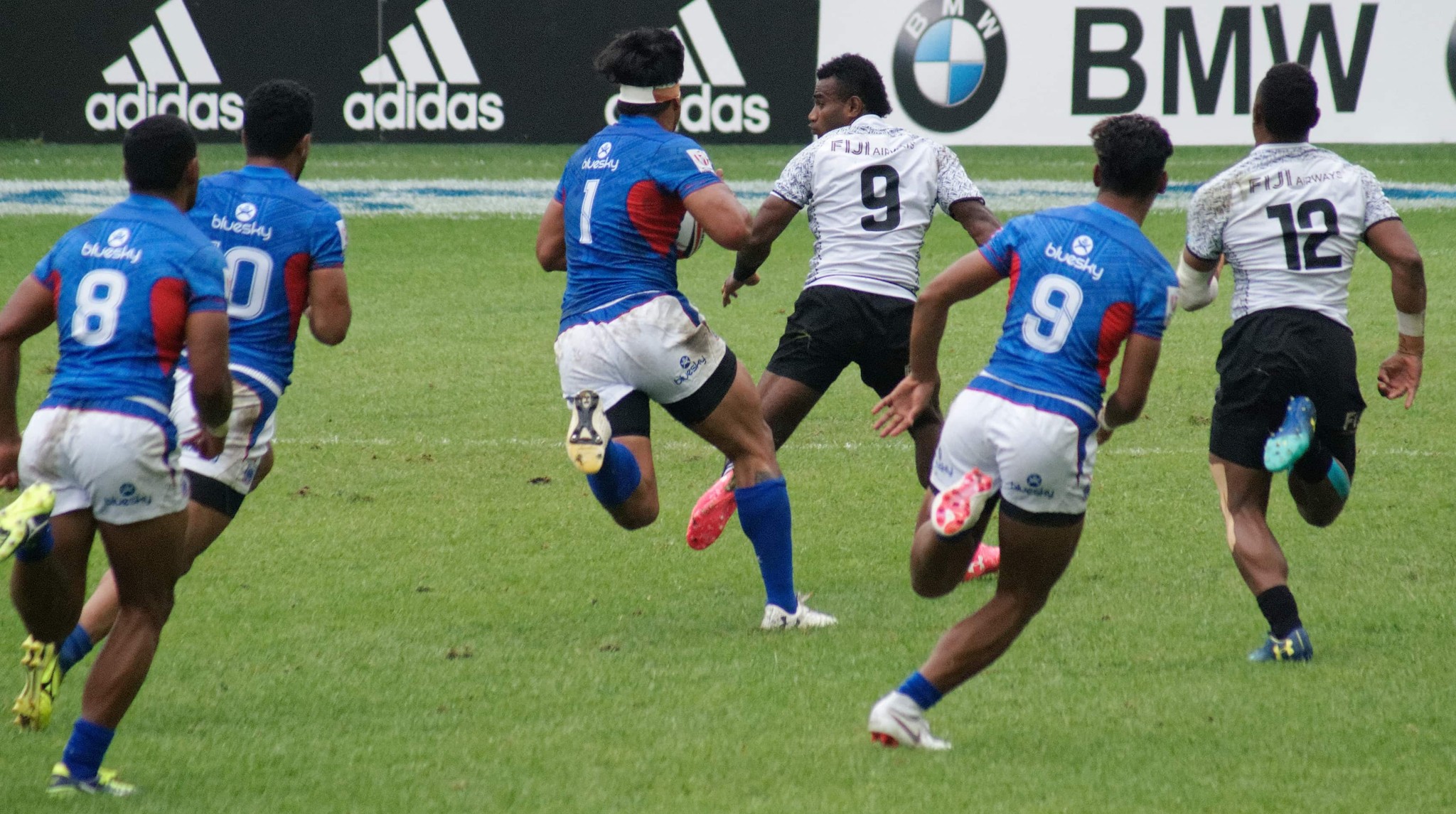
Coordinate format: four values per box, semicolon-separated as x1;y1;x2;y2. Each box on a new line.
870;118;1175;749
1;79;352;806
1175;66;1427;668
537;28;837;632
686;51;1000;586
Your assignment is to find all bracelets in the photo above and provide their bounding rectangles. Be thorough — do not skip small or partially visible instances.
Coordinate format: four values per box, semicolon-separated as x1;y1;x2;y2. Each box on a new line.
1397;310;1426;339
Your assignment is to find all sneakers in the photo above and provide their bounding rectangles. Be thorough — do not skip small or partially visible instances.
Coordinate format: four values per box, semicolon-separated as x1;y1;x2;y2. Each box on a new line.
0;480;56;563
45;761;135;800
565;388;612;475
686;467;738;550
12;634;63;735
867;691;952;752
1241;627;1313;664
930;465;992;537
1262;394;1317;473
962;542;1001;581
761;591;838;630
